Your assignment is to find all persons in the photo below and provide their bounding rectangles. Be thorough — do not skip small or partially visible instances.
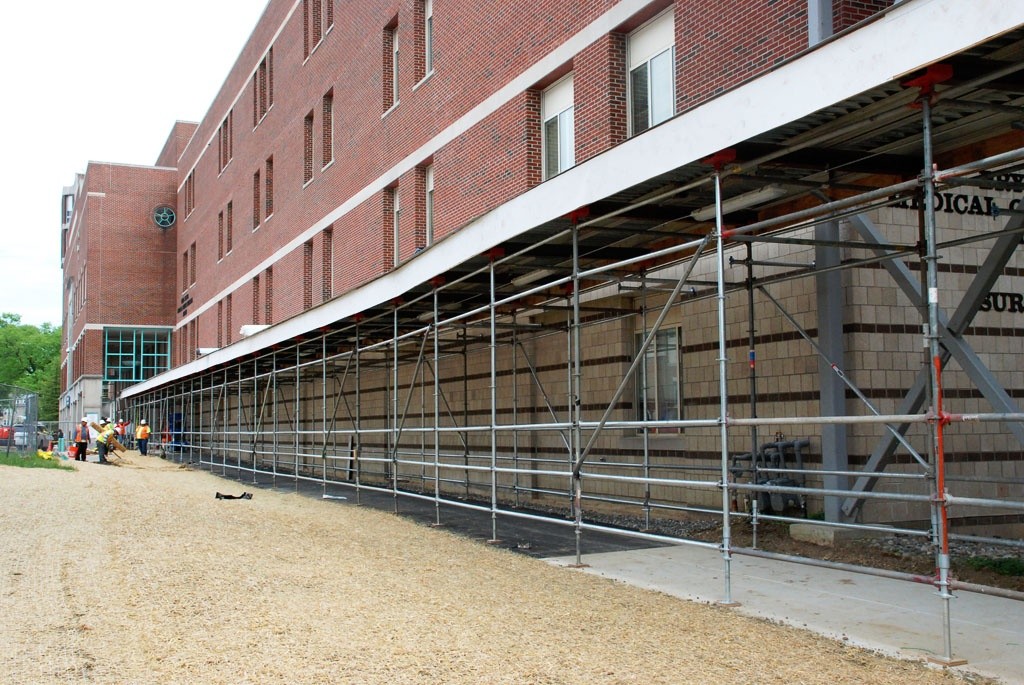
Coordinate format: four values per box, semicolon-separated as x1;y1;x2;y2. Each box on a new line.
71;417;91;461
51;429;64;441
161;427;172;451
95;416;131;464
135;419;151;456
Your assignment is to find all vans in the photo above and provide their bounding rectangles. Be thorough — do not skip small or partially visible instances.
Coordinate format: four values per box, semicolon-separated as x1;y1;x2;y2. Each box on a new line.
11;424;54;450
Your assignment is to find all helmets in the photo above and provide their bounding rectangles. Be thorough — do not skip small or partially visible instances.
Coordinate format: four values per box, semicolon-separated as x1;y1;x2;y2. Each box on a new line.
82;417;88;423
100;419;106;424
115;428;121;433
106;418;112;422
119;419;124;423
140;419;147;425
